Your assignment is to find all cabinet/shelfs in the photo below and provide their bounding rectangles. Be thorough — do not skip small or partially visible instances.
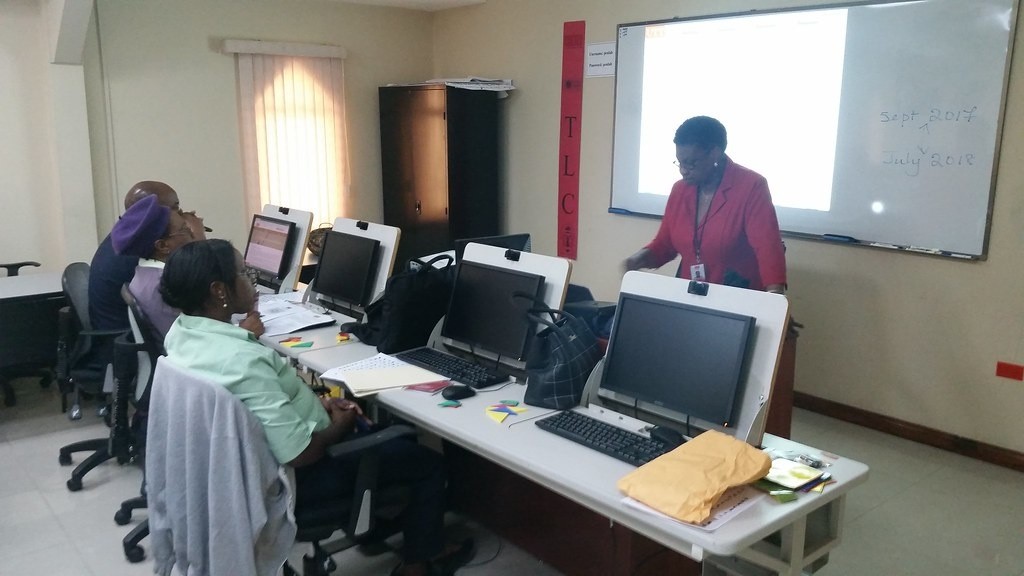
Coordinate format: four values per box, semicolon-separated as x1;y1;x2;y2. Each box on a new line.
378;84;498;276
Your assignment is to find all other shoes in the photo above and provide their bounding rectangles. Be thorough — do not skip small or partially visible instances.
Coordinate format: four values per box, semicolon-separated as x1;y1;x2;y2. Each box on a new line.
398;522;476;576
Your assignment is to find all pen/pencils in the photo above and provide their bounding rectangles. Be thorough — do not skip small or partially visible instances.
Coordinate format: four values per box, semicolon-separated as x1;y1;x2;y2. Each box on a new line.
242;315;265;320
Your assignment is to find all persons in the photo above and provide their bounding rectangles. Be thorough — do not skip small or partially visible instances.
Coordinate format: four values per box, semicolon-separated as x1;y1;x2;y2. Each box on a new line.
86;180;477;575
625;115;805;340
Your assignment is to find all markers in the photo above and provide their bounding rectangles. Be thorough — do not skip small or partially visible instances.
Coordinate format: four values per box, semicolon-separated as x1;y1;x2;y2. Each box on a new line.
789;451;818;467
609;208;631;214
823;234;860;243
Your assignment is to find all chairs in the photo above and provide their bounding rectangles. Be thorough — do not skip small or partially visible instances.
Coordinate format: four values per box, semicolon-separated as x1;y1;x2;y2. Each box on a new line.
113;280;167;563
59;262;131;492
158;355;422;576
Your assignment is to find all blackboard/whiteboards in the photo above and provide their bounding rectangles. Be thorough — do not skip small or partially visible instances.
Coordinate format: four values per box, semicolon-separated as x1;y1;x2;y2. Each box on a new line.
610;0;1020;264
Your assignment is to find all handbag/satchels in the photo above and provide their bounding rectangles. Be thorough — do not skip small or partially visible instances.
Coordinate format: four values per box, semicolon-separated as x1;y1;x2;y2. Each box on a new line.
507;290;601;410
366;254;456;353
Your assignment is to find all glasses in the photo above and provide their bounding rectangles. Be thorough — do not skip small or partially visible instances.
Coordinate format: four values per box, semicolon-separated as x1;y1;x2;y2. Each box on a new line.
673;147;715;171
236;267;260;282
161;224;195;239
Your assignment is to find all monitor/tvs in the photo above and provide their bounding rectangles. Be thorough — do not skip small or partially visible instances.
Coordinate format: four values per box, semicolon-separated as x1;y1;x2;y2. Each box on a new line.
599;292;757;426
311;230;380;308
243;215;296;280
440;260;546;361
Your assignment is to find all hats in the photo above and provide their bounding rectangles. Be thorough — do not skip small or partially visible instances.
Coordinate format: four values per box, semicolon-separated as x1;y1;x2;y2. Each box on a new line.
111;194;171;257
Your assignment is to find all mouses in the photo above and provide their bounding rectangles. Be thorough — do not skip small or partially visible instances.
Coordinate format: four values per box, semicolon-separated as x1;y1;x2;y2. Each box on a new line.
441;386;477;400
650;424;688;448
341;322;361;332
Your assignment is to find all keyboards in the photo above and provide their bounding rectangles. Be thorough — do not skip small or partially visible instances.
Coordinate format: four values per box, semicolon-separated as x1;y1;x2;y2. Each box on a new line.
537;410;678;466
395;348;510;389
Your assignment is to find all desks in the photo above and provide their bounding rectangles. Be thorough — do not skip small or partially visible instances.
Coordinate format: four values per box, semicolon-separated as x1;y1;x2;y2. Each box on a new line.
0;274;68;412
231;281;360;359
295;341;870;576
